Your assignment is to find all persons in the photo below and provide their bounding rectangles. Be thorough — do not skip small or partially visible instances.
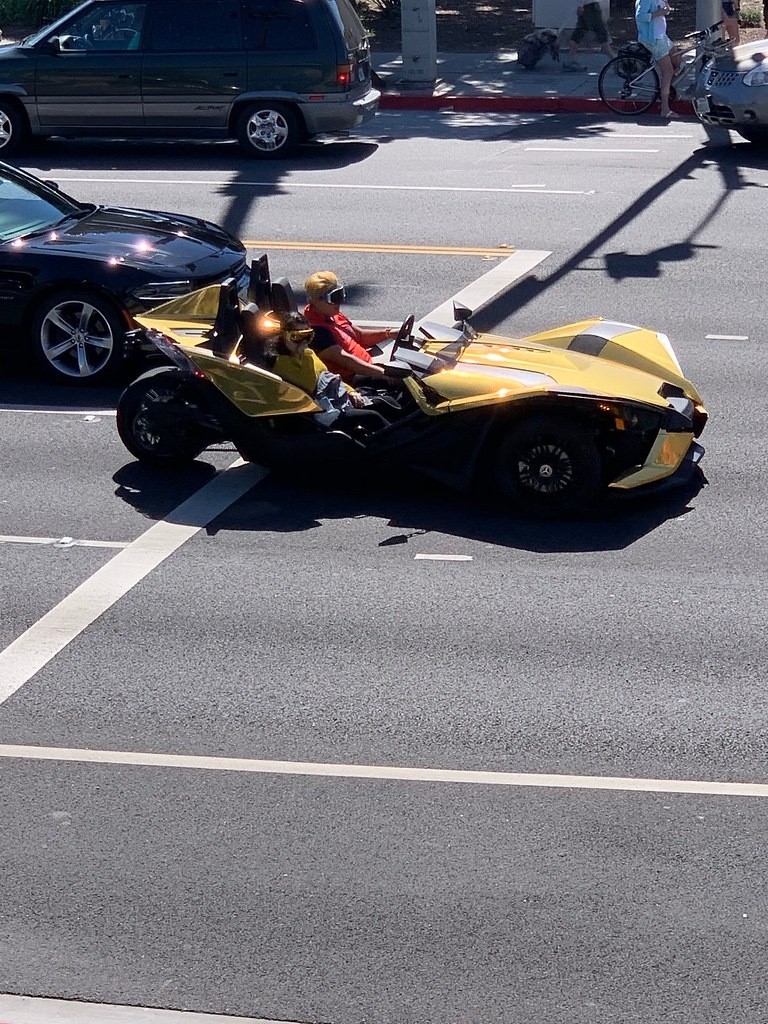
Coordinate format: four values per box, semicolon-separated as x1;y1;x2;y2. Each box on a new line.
562;0;626;72
263;310;404;436
634;0;683;120
762;0;768;39
302;270;413;401
721;0;741;46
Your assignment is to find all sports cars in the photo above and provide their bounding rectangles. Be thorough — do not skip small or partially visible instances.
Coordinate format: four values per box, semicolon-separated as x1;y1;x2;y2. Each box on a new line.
112;256;711;519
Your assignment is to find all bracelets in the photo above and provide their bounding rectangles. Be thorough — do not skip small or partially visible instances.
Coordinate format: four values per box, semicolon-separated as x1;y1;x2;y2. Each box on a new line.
385;328;391;339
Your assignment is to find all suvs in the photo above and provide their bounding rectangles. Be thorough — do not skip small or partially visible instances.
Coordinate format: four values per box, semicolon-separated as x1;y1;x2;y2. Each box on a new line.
0;1;387;157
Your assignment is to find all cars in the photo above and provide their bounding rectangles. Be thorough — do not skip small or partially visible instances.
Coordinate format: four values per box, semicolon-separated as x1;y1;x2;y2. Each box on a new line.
692;37;768;151
0;156;263;391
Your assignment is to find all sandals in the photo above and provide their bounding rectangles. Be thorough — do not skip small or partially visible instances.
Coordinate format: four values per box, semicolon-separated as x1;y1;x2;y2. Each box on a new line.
659;111;680;119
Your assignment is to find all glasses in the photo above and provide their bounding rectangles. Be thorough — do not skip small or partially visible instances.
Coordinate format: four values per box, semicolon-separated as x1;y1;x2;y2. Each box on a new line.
319;285;346;304
280;329;315;344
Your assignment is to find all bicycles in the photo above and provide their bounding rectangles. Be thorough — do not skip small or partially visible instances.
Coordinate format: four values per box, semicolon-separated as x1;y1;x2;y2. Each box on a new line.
597;18;731;117
